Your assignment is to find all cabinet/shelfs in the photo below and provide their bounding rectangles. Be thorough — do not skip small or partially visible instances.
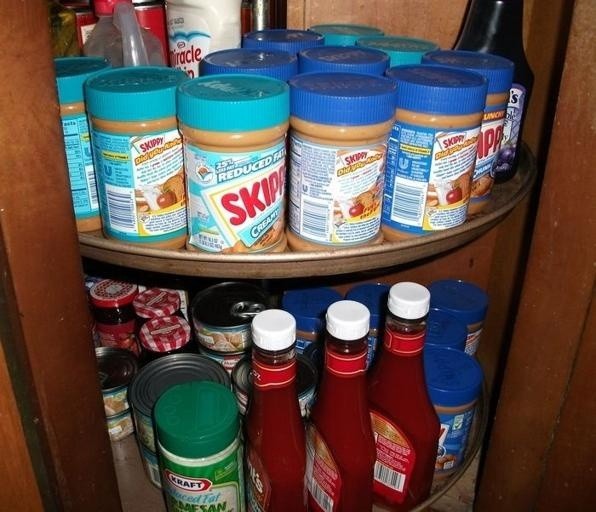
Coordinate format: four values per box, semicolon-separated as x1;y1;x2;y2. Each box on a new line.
0;0;595;511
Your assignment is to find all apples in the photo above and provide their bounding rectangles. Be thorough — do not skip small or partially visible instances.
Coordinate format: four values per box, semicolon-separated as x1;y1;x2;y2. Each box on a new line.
446;186;462;204
349;202;364;217
157;188;177;208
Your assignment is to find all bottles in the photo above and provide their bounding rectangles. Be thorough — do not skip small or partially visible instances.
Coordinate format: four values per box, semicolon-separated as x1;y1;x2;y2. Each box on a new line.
244;309;308;511
281;286;344;355
450;0;537;180
346;283;393;364
429;278;489;359
306;296;378;509
80;1;166;68
88;279;203;367
153;383;245;512
366;282;440;511
425;341;483;471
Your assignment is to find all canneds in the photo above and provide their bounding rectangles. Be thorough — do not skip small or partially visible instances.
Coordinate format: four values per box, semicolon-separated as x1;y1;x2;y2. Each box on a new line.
85;275;320;492
61;0;169;68
